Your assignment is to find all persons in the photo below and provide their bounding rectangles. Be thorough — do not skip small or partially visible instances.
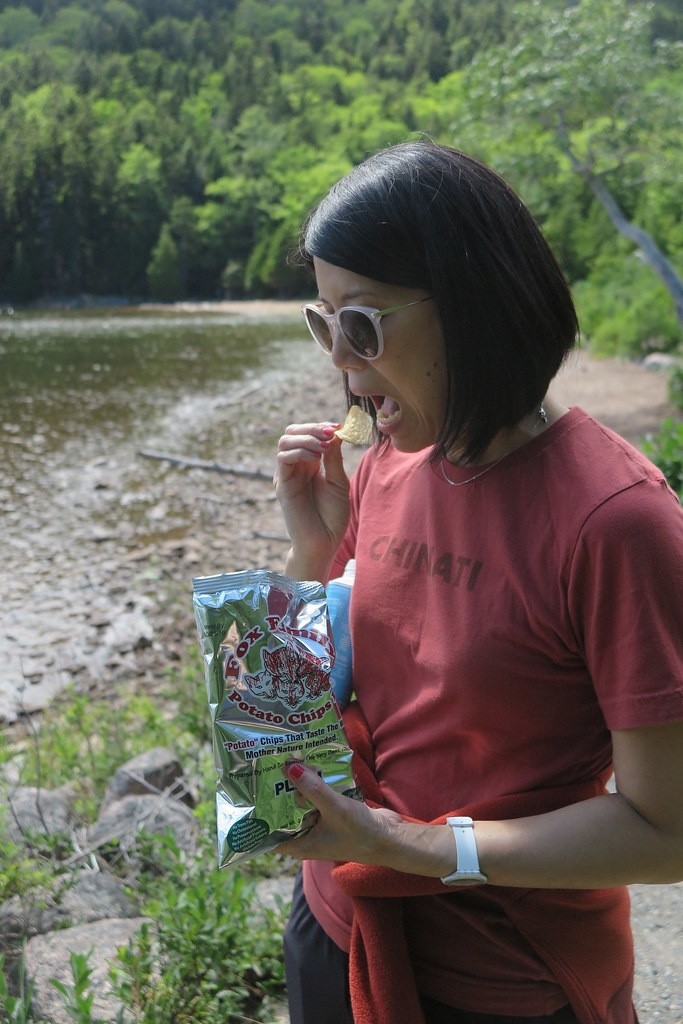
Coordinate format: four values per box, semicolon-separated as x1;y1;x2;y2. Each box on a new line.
273;142;683;1024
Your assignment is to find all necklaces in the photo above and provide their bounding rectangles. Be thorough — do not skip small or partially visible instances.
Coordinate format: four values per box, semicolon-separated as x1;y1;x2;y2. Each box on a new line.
441;400;548;486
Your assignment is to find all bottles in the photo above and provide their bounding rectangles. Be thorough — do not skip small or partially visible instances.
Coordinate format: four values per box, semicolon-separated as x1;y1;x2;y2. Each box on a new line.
323;558;356;714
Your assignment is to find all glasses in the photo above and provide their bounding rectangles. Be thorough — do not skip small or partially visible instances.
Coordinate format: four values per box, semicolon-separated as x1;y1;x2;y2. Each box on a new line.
301;293;435;362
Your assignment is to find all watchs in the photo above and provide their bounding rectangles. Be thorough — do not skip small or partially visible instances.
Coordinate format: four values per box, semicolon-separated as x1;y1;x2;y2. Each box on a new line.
440;816;487;887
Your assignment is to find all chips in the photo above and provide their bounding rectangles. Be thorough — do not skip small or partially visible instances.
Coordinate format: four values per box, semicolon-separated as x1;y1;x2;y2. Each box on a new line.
334;405;374;445
